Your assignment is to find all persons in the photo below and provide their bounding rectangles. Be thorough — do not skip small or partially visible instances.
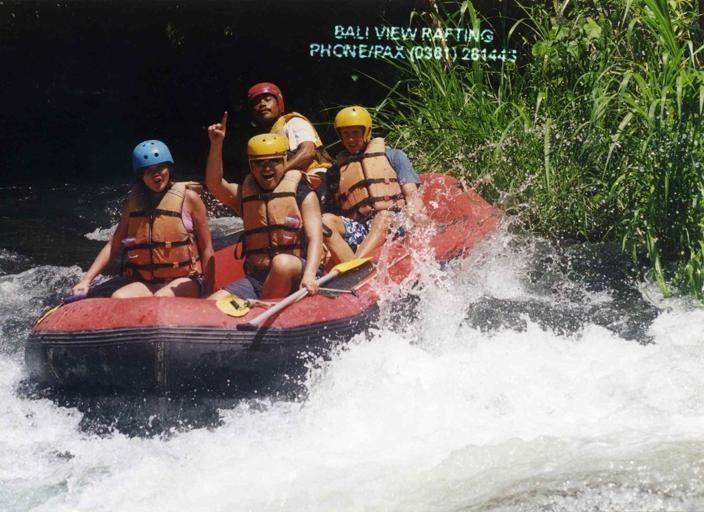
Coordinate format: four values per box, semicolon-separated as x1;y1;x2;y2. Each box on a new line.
322;105;422;265
246;81;335;203
205;111;328;301
69;140;215;299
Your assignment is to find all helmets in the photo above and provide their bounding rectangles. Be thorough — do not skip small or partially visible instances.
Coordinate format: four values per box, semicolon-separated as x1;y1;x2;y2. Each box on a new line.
131;139;174;176
246;82;284;112
334;105;372;144
247;133;290;174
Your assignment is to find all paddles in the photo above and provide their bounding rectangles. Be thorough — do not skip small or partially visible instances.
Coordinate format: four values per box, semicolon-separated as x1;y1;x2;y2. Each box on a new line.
216;297;276;316
236;258;372;331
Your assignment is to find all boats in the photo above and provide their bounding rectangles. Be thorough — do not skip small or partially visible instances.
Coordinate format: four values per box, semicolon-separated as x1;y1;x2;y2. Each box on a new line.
24;173;505;400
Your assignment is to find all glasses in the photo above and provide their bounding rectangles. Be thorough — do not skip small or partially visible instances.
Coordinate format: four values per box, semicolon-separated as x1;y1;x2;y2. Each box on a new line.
254;159;281;169
